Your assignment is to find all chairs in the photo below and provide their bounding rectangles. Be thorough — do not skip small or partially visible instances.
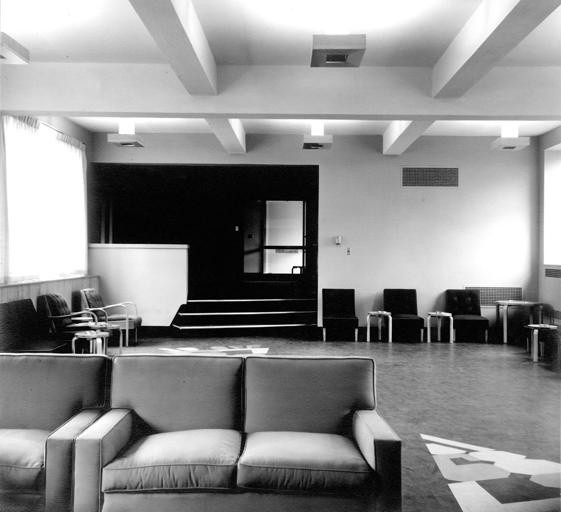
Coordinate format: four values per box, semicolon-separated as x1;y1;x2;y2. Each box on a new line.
445;290;490;343
383;289;424;343
322;289;358;342
1;288;142;354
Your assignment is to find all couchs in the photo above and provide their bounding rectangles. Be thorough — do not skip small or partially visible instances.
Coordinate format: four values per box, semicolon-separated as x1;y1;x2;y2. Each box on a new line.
71;353;402;512
0;352;111;512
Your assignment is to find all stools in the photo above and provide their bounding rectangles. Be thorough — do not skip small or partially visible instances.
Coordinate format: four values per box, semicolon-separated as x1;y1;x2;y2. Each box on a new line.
427;311;455;343
367;310;392;343
523;324;557;363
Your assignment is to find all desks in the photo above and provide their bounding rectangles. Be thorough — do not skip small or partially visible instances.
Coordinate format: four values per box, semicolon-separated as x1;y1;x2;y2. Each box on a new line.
495;299;544;345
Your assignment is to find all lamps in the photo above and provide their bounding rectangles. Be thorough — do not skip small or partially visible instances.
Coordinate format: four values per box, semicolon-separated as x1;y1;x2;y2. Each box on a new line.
302;134;332;153
106;133;143;147
309;34;365;68
489;138;531;155
